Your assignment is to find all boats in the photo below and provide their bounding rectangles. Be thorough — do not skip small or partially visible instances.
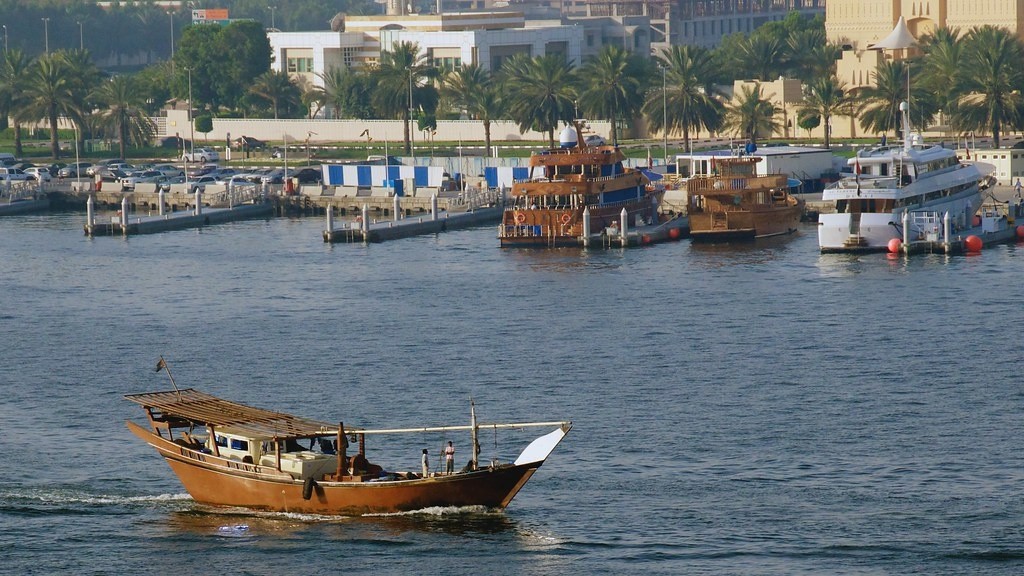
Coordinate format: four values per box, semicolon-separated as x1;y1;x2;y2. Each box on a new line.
816;101;998;252
494;122;665;249
127;352;575;520
685;154;807;241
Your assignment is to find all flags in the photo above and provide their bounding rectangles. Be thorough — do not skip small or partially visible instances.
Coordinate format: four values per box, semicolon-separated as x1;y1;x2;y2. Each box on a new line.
856;159;860;197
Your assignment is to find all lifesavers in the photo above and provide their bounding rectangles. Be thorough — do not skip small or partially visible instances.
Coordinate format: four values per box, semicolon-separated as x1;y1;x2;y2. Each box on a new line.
355;217;363;225
562;214;571;224
515;212;525;222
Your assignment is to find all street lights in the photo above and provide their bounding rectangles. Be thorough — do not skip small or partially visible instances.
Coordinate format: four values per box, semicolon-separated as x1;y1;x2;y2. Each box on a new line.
405;65;414;164
659;64;667;164
903;60;911;137
267;6;278;28
166;10;176;62
76;20;83;60
41;17;51;54
2;24;7;55
183;66;195;163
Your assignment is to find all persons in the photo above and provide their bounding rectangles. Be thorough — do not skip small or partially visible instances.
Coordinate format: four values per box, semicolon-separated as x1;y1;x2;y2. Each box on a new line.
58;169;64;185
610;219;618;235
881;133;886;146
445;441;454;475
1014;178;1024;197
531;202;537;210
738;149;745;162
422;449;429;479
36;172;42;186
272;149;282;159
354;216;363;228
6;174;11;188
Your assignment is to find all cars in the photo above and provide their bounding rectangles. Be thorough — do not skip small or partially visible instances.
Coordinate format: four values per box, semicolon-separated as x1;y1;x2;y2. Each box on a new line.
583;135;607;147
232;137;266;149
181;147;219;164
0;150;324;194
